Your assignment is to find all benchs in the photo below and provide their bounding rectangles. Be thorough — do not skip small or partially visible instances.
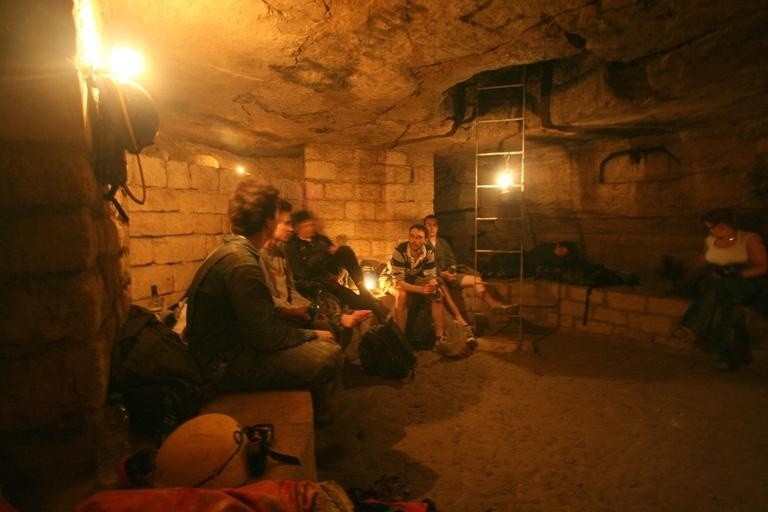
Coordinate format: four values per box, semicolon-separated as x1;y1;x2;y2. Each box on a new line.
376;273;755;360
197;386;319;487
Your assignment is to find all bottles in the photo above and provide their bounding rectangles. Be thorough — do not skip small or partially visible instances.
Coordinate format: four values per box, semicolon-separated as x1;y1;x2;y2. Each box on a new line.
146;285;164;320
99;392;131;478
303;288;323;329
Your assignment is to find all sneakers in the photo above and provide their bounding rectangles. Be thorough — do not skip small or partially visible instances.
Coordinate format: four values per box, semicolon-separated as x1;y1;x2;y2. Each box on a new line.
464;323;475;344
432;336;460;357
491;304;520;314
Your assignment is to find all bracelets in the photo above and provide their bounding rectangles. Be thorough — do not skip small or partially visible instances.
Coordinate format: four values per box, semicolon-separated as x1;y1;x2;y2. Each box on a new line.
740;268;744;280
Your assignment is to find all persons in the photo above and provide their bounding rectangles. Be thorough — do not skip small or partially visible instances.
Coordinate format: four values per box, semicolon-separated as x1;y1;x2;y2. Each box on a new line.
424;213;521;347
259;197;374;329
387;224;455;357
184;177;348;431
660;206;768;377
282;208;391;325
478;239;576;280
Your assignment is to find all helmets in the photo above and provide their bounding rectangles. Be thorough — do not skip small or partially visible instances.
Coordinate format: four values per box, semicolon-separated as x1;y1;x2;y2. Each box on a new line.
156;412;251;487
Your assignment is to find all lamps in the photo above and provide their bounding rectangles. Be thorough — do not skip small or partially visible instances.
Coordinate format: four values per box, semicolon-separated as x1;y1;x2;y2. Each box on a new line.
358;261;378;291
495;152;518;196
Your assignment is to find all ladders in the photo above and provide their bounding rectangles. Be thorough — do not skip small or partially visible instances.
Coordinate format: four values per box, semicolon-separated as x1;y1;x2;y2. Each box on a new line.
466;64;535;353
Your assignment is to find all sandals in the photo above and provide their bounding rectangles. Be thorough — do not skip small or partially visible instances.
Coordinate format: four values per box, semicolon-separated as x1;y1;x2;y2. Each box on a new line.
715;358;734;373
666;327;700;346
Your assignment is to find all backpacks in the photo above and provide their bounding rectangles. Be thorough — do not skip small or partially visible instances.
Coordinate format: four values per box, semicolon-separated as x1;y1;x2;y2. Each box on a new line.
107;303;215;437
478;241;624;290
357;320;416;380
